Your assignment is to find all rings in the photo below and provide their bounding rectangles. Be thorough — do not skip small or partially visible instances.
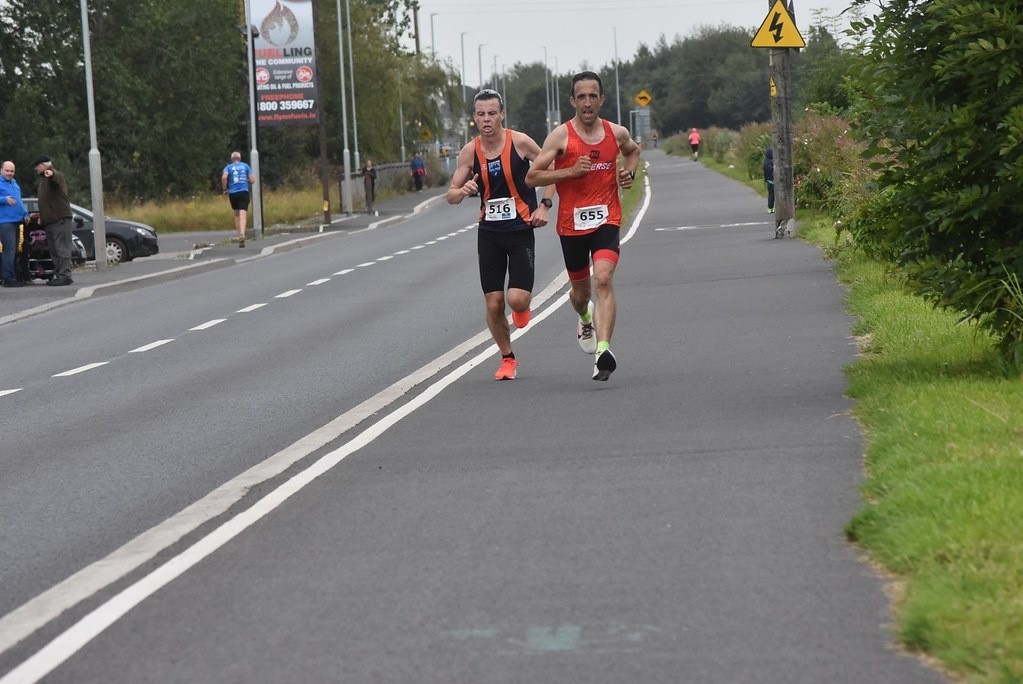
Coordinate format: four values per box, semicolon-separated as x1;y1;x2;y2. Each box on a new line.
622;181;625;185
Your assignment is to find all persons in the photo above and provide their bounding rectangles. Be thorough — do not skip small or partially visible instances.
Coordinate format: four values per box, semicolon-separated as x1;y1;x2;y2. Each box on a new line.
360;160;376;205
221;151;254;248
30;155;73;286
410;151;427;192
763;149;775;213
636;129;659;149
523;71;642;382
445;88;556;383
0;160;30;288
688;128;701;161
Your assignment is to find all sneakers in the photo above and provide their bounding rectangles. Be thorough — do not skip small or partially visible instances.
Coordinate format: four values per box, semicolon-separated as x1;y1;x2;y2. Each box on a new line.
592;349;617;381
577;300;597;354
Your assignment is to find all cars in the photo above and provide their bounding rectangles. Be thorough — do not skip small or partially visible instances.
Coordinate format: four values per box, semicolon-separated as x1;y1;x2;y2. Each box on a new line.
20;197;160;265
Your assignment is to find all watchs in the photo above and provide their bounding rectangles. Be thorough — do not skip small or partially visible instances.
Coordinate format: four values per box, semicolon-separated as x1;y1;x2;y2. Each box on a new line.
541;199;552;207
630;171;635;180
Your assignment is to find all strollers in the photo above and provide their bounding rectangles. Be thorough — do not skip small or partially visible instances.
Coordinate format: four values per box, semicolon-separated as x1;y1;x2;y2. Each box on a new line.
23;211;56;281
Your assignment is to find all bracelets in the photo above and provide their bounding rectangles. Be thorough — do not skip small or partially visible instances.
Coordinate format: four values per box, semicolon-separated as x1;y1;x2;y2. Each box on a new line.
222;190;226;191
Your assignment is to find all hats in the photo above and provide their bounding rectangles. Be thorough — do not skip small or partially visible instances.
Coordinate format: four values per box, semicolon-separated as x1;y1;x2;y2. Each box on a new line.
32;156;50;166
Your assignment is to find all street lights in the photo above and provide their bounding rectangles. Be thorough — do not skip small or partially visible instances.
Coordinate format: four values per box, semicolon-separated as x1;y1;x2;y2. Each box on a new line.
479;44;484;90
460;33;467;102
430;14;437;54
237;26;266;231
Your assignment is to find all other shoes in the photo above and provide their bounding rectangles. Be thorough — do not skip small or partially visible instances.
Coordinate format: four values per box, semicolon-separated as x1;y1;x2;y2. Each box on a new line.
46;274;73;286
239;236;245;248
512;307;531;328
2;280;24;287
495;358;520;380
767;208;775;214
